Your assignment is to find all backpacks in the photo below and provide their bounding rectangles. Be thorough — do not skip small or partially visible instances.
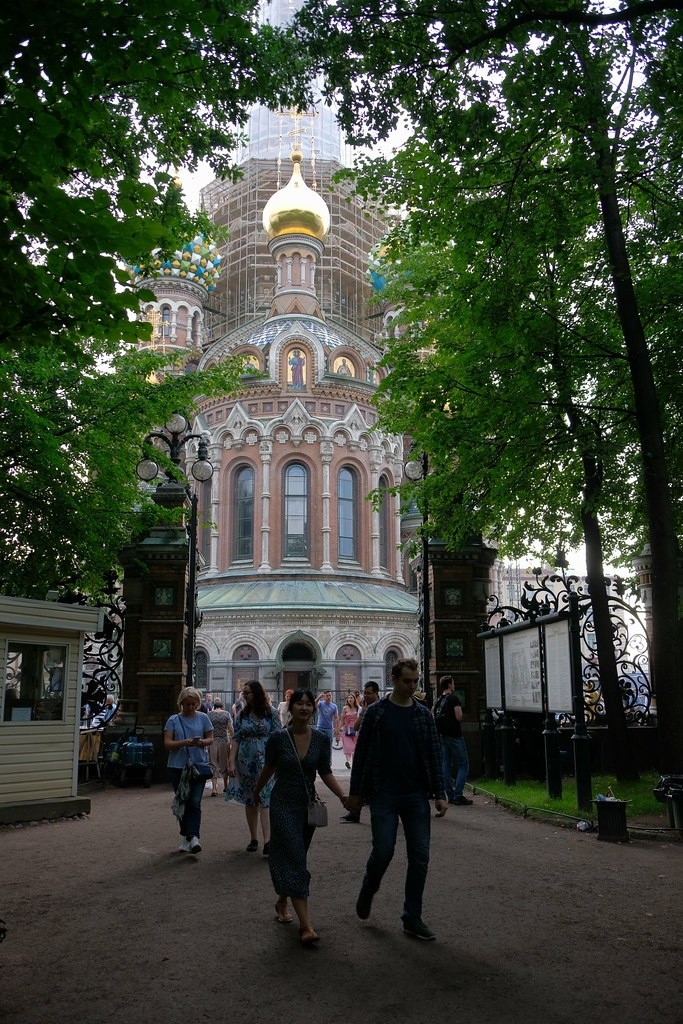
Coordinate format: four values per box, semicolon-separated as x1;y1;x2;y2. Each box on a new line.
434;694;451;730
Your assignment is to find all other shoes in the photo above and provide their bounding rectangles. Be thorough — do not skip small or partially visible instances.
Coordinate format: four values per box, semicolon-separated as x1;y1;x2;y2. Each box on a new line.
211;792;219;797
247;840;258;851
177;836;202;855
262;841;270;855
448;796;473;805
339;813;361;824
345;762;351;769
223;789;227;792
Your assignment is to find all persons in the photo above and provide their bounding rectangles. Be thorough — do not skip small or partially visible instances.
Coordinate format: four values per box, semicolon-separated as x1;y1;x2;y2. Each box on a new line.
6;688;19;700
254;687;361;943
228;681;281;854
435;676;473;805
80;694;122;730
197;681;380;824
164;686;214;854
347;659;449;939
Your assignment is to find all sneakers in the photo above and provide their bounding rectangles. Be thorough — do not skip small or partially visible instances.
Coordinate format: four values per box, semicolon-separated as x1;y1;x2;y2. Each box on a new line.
356;886;375;920
403;917;437;940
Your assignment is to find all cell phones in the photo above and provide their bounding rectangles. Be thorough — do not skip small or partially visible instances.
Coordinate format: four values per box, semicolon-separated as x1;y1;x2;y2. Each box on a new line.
193;737;200;741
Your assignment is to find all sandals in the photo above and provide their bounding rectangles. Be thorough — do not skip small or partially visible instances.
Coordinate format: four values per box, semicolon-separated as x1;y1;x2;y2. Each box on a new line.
298;927;319;944
274;902;294;922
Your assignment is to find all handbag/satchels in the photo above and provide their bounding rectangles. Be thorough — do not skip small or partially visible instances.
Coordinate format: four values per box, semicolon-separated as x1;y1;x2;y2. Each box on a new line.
308;799;328;827
345;727;356;737
193;762;214;780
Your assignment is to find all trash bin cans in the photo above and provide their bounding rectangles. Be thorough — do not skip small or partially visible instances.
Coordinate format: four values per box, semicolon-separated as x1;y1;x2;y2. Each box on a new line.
589;799;634;843
660;775;683;829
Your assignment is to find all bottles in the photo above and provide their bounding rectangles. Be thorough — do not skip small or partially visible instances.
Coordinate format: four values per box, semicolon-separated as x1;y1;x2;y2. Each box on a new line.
103;736;154;764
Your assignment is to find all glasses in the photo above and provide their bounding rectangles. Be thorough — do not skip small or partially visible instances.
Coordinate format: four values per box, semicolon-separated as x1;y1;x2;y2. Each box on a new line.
242;691;253;696
